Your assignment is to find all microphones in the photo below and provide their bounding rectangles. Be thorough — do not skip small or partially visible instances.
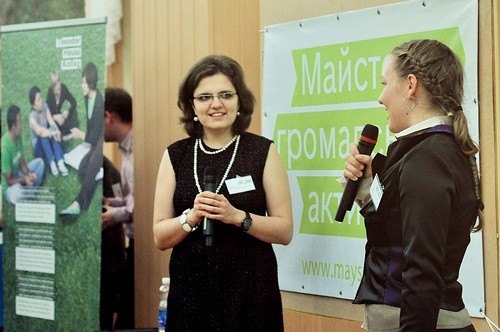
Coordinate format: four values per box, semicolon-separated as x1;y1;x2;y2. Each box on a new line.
202;165;219;248
335;124;378;221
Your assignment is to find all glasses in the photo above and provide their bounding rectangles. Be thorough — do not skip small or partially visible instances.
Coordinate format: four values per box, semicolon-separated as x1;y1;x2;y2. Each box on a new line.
192;92;237;102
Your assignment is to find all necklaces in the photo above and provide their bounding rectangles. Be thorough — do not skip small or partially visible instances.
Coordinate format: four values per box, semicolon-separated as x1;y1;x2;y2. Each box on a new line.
193;134;241;194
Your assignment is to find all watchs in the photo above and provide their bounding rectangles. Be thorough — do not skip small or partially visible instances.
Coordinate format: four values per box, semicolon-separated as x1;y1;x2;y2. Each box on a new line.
238;211;253;232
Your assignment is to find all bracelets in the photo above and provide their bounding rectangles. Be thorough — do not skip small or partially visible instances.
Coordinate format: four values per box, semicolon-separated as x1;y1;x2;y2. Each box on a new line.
181;208;201;232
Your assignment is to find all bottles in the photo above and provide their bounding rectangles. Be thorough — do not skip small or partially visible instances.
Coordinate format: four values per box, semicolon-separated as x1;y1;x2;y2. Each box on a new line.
157;277;170;332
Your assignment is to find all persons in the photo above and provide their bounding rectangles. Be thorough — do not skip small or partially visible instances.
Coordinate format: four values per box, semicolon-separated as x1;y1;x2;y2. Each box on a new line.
345;38;485;332
0;63;105;216
152;55;293;332
102;86;136;332
